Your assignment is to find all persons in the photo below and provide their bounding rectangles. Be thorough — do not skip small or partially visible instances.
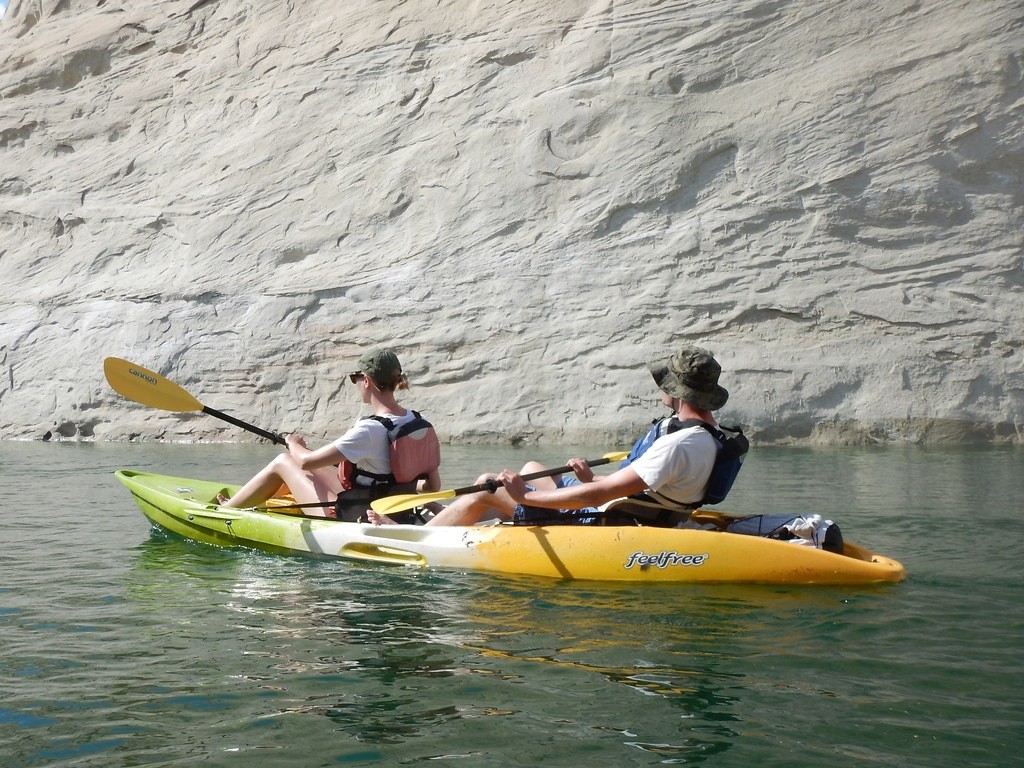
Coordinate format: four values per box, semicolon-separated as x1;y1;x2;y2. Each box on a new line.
217;349;441;518
367;345;728;526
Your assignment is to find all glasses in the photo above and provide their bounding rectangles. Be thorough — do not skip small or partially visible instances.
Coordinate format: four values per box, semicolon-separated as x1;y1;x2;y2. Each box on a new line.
349;370;384;394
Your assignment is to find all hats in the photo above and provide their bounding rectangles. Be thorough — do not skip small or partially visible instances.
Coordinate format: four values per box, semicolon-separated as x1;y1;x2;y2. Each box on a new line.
647;343;729;411
355;348;402;384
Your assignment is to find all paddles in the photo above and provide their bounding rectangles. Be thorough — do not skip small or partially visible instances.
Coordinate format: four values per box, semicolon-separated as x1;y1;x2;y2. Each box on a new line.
103;356;342;468
368;451;630;517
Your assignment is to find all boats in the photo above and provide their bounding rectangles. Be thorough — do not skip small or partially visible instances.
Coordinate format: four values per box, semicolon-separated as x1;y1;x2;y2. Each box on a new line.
115;465;905;588
